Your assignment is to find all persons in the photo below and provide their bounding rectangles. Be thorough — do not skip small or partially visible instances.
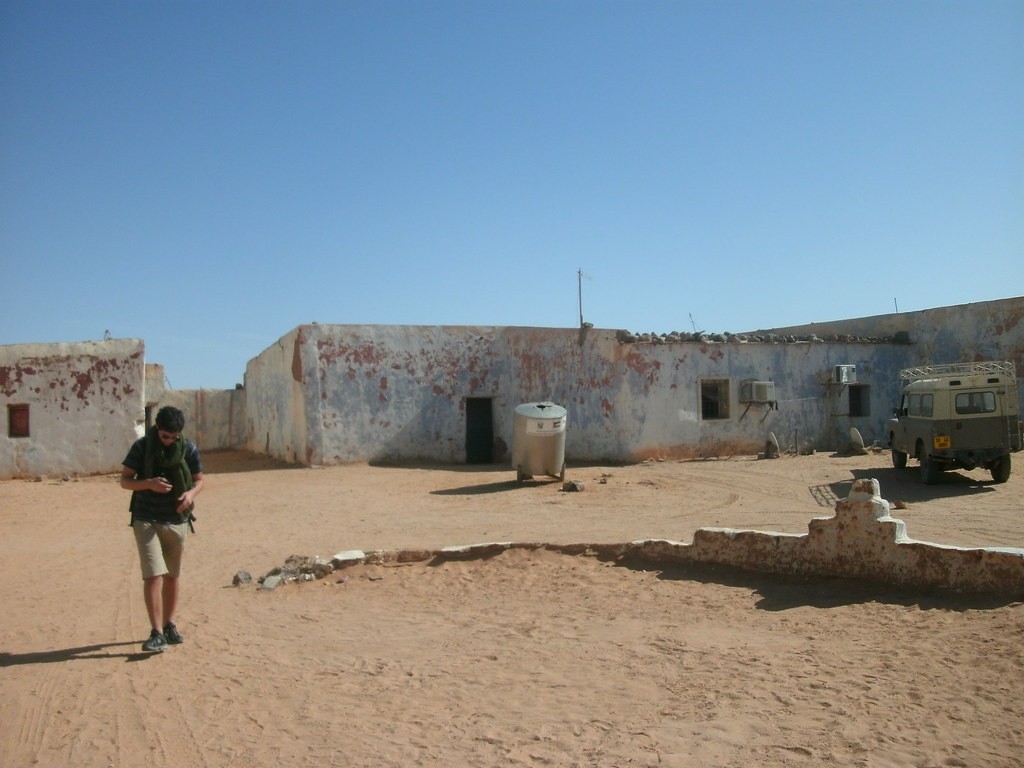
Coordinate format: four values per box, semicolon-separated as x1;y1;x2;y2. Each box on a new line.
120;408;203;652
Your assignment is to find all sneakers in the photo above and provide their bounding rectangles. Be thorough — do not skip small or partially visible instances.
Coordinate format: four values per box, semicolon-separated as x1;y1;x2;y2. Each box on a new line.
142;628;167;652
163;622;183;643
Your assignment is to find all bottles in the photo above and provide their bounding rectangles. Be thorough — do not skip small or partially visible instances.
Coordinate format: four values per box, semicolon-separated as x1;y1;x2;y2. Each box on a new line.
314;555;320;564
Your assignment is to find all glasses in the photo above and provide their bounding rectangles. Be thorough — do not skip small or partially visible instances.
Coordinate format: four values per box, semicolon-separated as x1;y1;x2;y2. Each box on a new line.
160;429;180;440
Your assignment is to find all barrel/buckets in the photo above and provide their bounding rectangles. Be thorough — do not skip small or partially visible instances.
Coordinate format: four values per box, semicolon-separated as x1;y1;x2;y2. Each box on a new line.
511;402;567;475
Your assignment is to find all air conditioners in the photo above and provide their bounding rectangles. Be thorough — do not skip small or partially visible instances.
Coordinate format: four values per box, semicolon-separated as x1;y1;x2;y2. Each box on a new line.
833;364;857;383
751;381;775;402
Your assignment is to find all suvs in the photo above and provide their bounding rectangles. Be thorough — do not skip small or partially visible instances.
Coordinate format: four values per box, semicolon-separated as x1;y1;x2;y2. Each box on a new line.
887;362;1024;486
